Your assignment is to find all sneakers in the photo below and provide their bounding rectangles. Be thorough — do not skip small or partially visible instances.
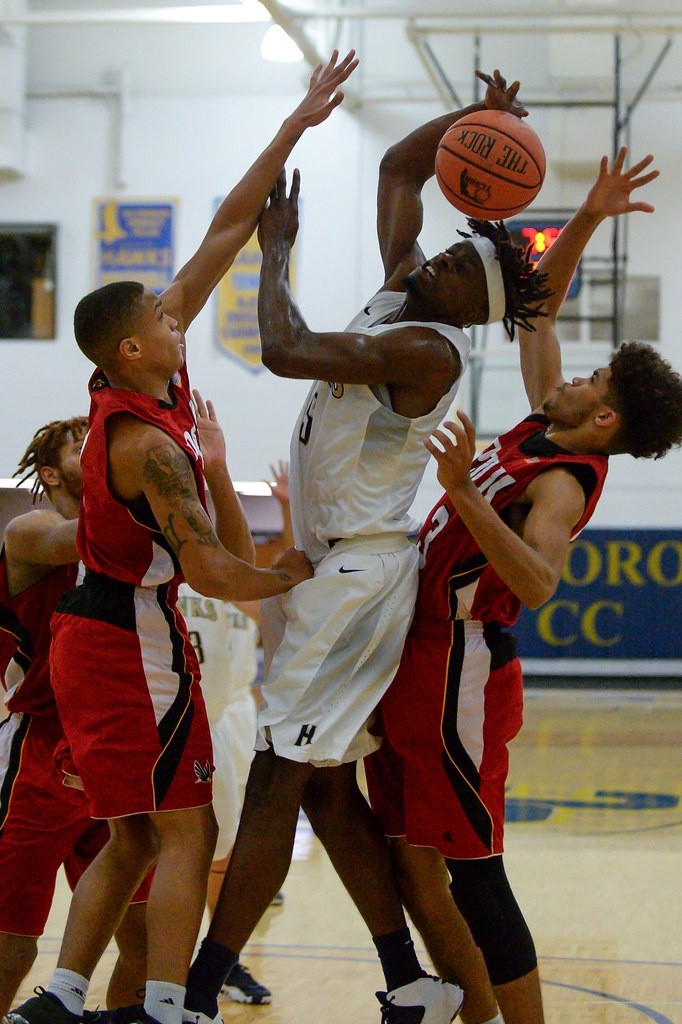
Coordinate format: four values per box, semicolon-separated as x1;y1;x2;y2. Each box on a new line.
2;985;106;1024
220;962;272;1004
182;1007;225;1024
375;970;465;1024
104;1003;162;1024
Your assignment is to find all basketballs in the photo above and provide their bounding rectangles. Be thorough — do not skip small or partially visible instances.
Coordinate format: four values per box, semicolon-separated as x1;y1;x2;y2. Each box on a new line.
433;108;550;222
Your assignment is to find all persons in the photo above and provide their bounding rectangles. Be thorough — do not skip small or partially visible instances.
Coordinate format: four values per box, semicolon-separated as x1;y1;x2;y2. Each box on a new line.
0;50;682;1024
